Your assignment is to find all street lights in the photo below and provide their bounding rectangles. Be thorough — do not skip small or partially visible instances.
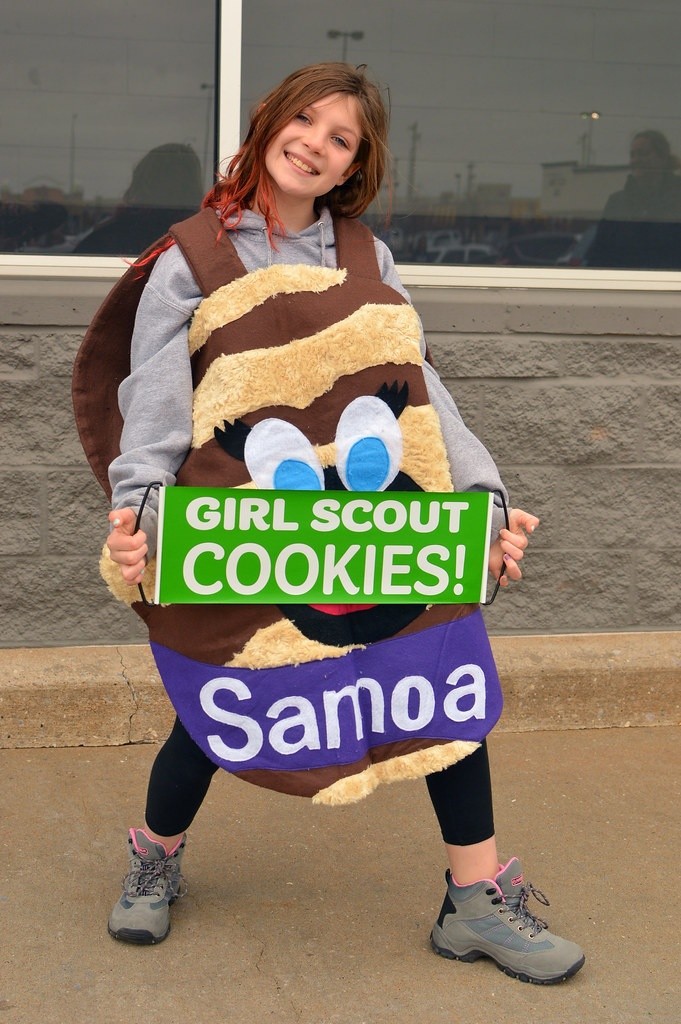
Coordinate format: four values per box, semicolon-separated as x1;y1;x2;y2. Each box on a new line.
324;28;364;64
580;111;600;167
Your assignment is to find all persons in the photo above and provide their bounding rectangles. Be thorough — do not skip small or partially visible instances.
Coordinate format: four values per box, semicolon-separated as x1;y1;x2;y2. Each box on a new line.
73;144;203;256
585;128;681;270
107;62;585;985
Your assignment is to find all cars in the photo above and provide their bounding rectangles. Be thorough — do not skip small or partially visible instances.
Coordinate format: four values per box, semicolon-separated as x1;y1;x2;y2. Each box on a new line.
496;230;598;267
434;244;497;266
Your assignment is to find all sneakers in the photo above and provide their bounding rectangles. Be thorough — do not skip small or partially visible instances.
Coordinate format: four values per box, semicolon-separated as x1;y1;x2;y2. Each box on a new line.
429;857;585;984
106;828;187;945
